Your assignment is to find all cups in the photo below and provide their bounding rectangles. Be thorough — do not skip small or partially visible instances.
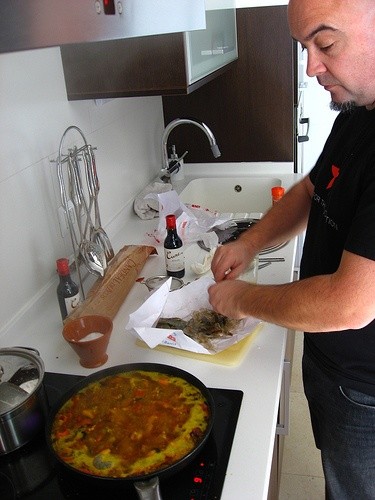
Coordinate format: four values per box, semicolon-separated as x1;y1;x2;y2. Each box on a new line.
63;316;113;369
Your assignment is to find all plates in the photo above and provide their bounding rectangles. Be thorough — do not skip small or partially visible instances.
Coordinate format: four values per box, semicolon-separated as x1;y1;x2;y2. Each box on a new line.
197;218;292;255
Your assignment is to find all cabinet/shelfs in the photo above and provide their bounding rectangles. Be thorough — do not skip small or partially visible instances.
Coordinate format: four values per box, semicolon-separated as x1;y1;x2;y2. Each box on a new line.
161;5;310;174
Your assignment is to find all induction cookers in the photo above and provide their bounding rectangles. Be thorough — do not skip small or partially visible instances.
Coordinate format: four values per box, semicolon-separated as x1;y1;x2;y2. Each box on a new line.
0;373;243;500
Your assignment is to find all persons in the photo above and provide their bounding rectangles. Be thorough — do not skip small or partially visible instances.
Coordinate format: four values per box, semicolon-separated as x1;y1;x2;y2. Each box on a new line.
207;0;375;500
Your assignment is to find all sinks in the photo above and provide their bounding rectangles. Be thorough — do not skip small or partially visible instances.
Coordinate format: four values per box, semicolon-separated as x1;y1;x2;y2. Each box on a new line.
178;177;282;219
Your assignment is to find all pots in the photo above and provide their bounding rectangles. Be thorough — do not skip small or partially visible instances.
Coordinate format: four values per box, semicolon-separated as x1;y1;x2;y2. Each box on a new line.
45;363;214;500
1;346;50;456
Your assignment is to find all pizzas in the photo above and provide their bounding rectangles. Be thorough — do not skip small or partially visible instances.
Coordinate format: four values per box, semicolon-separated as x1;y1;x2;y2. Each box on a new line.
51;371;210;478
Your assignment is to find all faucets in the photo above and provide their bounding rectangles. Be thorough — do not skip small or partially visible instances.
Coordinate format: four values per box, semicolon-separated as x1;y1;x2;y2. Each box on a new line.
160;116;221;169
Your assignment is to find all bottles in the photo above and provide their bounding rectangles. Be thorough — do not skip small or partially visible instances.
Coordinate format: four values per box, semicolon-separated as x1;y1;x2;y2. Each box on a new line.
163;213;186;279
55;258;82;320
271;185;285;207
